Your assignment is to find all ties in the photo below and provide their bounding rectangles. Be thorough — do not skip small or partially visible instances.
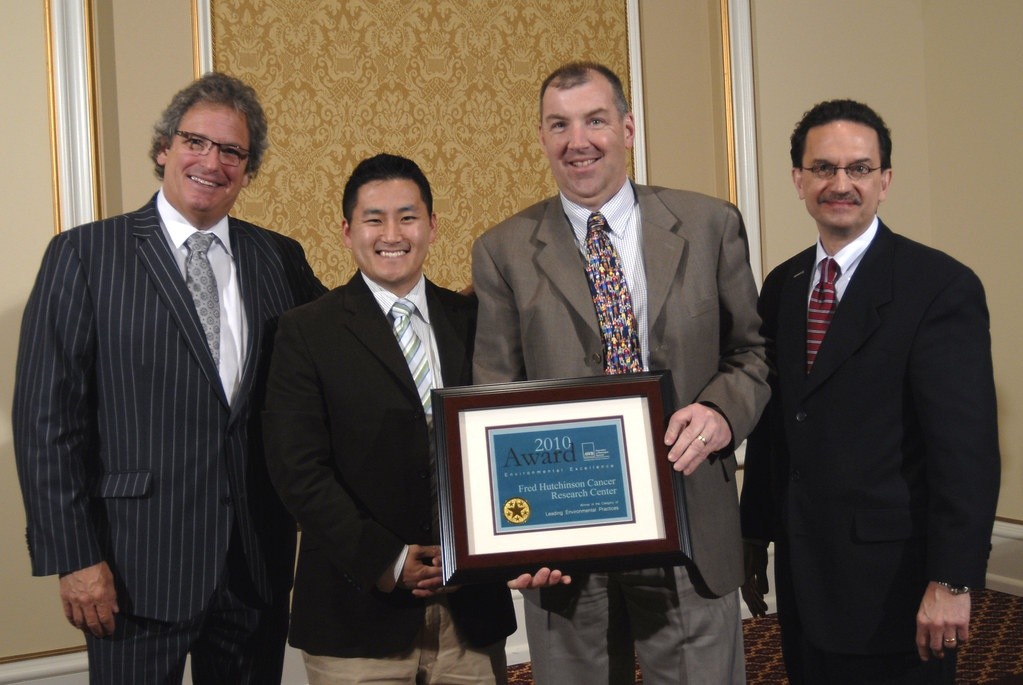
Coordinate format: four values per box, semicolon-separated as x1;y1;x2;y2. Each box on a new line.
389;296;436;472
185;231;220;371
582;210;643;375
805;256;841;374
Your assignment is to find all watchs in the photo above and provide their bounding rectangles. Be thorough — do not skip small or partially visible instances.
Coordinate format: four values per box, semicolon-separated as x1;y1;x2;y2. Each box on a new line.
934;581;970;594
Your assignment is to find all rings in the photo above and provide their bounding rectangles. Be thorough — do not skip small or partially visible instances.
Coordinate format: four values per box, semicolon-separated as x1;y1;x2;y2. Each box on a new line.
944;638;957;642
696;435;707;446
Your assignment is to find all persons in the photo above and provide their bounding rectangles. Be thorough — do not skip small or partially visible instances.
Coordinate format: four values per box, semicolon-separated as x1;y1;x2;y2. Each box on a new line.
471;59;770;685
11;73;329;685
264;153;518;685
738;99;1000;685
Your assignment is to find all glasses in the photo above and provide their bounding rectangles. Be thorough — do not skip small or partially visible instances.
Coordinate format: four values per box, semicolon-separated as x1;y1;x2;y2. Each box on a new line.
172;127;251;165
801;161;882;180
